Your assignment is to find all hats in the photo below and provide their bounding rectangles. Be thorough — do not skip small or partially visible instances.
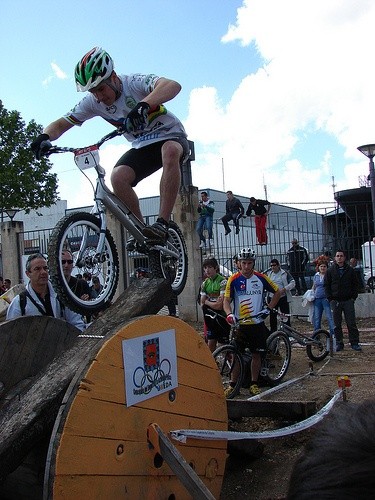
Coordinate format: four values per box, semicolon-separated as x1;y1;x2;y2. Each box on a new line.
92;277;99;282
250;197;256;202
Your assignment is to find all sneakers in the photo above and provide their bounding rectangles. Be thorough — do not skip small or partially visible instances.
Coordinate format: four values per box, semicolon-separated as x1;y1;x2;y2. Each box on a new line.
210;238;214;246
199;242;207;247
336;345;344;352
236;228;239;234
143;223;168;238
224;384;236;397
351;345;362;352
249;385;262;395
266;359;275;368
126;223;150;250
225;229;231;235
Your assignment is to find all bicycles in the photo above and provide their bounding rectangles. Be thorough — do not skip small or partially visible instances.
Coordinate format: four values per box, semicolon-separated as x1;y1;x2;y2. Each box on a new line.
43;122;190;316
204;307;280;398
262;308;332;385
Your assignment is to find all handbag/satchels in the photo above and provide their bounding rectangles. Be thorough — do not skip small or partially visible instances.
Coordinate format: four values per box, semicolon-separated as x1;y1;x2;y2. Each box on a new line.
203;304;231;330
241;353;270;388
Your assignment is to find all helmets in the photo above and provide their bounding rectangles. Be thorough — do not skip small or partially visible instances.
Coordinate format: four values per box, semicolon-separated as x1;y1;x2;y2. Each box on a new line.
75;274;82;278
83;272;92;277
75;47;113;92
136;267;146;276
238;248;256;259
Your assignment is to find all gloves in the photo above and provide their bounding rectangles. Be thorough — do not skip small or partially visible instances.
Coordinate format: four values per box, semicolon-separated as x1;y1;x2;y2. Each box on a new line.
226;313;237;325
257;307;271;321
30;134;49;161
122;102;151;139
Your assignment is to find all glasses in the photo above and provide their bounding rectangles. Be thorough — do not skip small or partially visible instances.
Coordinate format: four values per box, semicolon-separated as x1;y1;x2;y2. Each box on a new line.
291;242;297;244
61;260;74;264
270;264;278;267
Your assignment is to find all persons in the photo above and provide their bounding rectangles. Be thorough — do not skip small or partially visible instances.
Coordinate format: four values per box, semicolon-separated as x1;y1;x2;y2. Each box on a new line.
245;197;271;246
30;54;190;245
312;250;366;352
197;256;296;362
0;249;177;335
221;191;244;235
223;249;282;398
197;192;215;248
285;238;309;296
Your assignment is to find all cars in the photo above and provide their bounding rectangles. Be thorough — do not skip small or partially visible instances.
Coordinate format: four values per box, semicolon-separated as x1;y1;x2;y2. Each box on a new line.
262;261;324;297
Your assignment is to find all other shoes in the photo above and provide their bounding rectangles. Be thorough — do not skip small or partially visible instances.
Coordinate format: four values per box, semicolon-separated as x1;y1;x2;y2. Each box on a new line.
259;242;266;244
87;322;93;325
